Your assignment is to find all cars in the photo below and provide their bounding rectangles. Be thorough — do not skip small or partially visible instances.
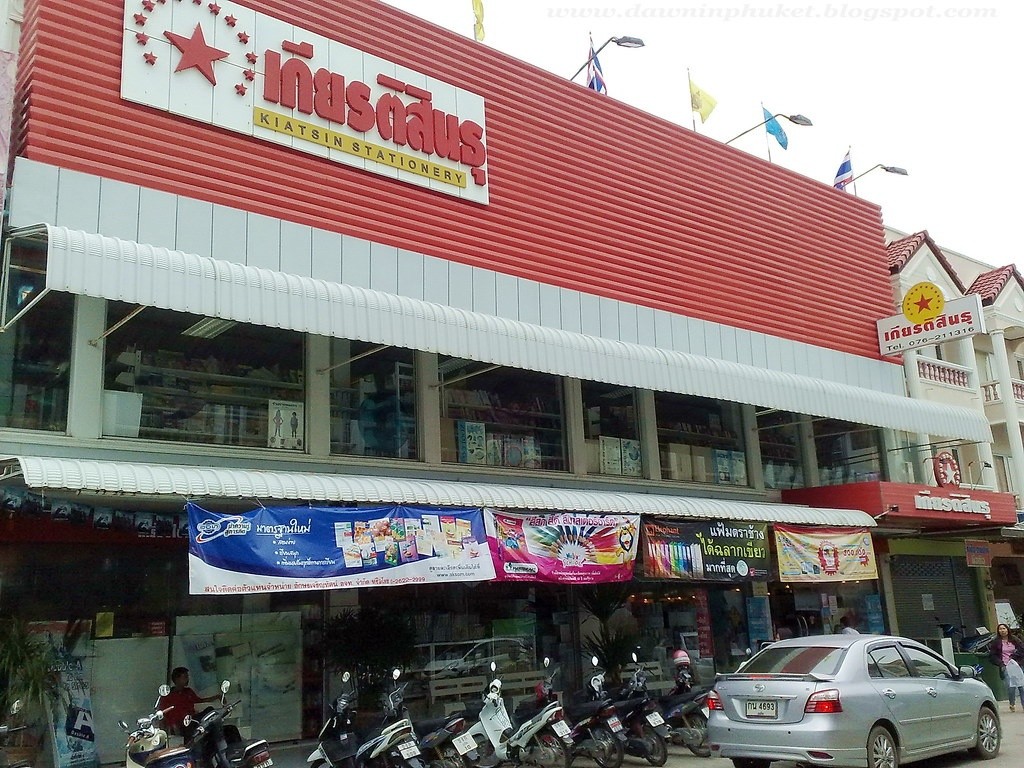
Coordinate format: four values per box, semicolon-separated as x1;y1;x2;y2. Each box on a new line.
705;626;1003;768
425;638;530;679
652;632;746;663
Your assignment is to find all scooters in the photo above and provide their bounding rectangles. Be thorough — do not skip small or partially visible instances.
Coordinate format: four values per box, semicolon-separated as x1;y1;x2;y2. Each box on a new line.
666;666;694;695
308;672;424;768
512;657;629;768
0;700;31;768
117;684;192;768
380;668;481;768
617;652;711;758
936;616;995;653
184;679;273;768
583;655;671;767
462;662;575;768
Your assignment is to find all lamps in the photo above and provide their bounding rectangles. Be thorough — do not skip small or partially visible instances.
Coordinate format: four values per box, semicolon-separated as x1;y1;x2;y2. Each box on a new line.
569;36;646;82
725;113;814;145
840;163;909;190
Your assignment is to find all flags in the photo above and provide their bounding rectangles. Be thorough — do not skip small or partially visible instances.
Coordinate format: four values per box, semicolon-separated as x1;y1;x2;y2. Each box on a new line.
833;150;854;193
688;71;718;125
473;0;485;42
762;107;788;150
587;35;607;96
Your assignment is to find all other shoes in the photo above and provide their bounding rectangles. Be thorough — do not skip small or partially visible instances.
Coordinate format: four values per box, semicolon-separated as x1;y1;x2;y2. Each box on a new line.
1010;705;1015;712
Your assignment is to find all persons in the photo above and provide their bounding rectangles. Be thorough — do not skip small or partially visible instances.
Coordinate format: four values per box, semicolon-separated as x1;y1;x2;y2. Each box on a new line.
273;410;284;437
775;628;792;642
160;667;227;747
290;412;298;438
833;615;860;634
989;624;1024;711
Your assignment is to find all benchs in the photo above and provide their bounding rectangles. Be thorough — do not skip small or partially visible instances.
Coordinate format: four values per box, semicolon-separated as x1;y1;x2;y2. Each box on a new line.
496;669;563;716
424;676;491;719
618;662;676;697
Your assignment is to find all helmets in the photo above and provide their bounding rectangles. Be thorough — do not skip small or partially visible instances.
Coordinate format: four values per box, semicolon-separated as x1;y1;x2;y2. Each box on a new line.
673;650;690;666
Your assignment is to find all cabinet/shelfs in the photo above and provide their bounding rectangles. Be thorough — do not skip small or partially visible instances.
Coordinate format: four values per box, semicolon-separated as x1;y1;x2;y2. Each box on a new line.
119;350;803;494
